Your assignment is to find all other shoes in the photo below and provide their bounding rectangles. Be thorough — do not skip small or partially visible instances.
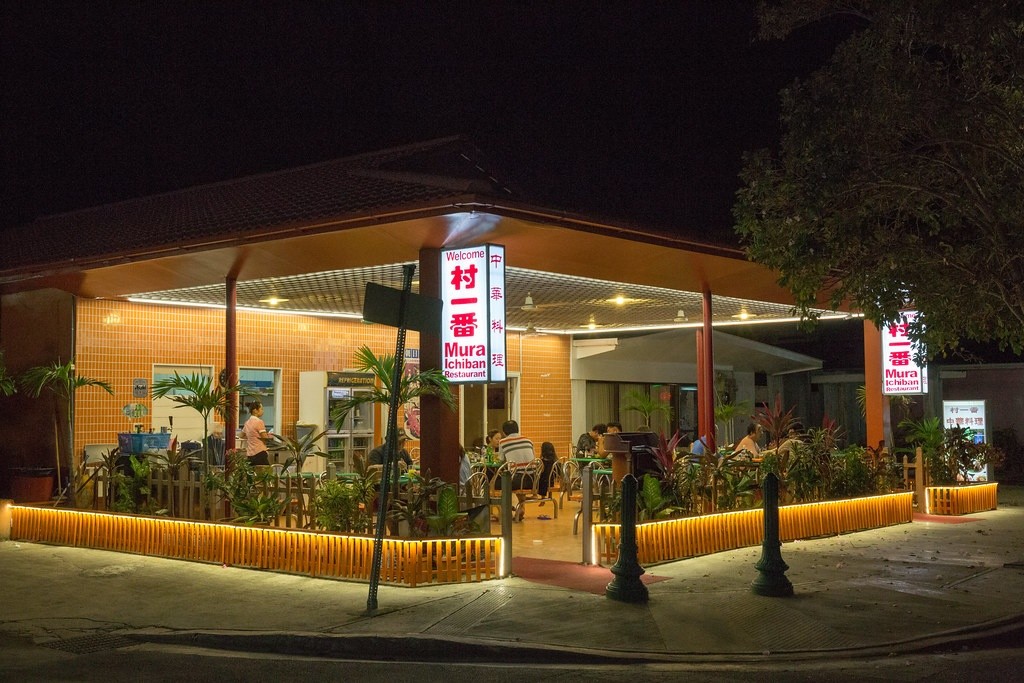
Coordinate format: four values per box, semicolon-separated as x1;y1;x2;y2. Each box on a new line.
512;506;517;512
538;497;545;507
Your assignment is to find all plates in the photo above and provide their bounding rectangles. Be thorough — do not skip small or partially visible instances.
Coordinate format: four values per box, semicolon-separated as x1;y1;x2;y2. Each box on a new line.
404;419;421;441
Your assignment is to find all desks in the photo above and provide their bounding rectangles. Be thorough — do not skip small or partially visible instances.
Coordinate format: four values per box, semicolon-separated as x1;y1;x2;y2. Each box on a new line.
470;462;503;485
592;468;614;517
267;471;360;528
373;477;420;520
570;457;609;470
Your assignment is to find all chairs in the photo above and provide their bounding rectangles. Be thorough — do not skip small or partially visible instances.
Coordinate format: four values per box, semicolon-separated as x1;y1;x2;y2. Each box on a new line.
407;446;612;536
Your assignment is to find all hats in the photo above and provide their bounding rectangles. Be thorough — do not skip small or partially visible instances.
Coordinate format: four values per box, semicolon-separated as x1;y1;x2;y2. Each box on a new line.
386;427;413;441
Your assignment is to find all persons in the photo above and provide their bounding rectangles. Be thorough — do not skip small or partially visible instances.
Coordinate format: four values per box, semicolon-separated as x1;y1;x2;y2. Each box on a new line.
481;430;503;490
778;423;813;471
576;423;652;483
500;420;536;521
366;427;414;537
459;442;474;489
734;422;777;459
539;442;560;505
691;424;718;465
240;401;274;466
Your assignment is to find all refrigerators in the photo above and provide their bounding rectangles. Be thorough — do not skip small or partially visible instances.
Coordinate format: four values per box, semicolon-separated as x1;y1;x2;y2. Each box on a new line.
298;370;375;511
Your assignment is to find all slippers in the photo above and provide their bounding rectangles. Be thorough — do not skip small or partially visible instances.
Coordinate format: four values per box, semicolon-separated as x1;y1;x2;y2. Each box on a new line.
537;514;551;520
512;516;524;521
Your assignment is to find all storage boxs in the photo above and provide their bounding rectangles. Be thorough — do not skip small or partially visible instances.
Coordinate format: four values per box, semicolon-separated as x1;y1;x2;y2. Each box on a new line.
118;432;171;454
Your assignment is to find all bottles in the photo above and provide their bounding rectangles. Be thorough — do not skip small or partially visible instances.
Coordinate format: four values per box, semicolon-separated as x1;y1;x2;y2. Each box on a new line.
327;405;365;461
482;446;493;463
